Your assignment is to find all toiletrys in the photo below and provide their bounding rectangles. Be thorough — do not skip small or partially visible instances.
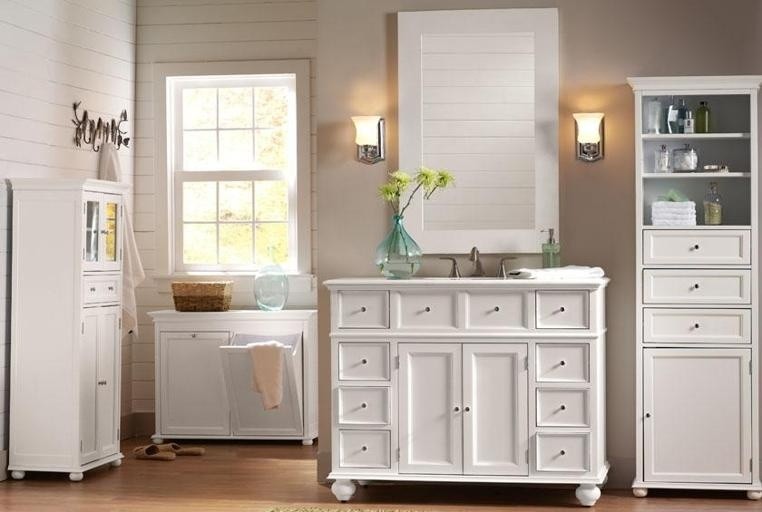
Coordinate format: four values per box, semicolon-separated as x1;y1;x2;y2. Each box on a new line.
542;228;562;268
646;96;712;134
683;110;694;134
655;143;671;172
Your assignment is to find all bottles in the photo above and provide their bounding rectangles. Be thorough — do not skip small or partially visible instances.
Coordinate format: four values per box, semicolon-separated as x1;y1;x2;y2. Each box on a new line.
646;95;711;133
99;143;122;181
653;143;698;173
702;182;724;226
253;269;290;310
541;229;561;269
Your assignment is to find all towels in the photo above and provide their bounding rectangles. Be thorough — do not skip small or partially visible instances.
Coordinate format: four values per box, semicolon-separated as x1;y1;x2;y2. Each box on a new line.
646;196;699;231
510;265;606;278
97;141;144;341
249;341;284;410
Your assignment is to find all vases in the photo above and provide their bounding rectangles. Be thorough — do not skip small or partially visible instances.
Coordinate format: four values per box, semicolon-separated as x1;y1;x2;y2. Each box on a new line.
253;244;289;312
374;214;423;281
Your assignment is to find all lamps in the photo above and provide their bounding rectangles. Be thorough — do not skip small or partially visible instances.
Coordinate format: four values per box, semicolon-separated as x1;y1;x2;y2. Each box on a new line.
348;114;385;164
572;110;606;163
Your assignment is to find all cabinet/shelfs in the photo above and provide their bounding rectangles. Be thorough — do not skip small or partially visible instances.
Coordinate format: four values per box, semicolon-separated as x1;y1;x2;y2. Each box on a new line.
320;277;617;509
147;309;318;446
7;172;129;481
625;72;762;505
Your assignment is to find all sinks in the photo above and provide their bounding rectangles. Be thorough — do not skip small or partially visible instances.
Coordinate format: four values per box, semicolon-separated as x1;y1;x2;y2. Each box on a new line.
414;275;534;282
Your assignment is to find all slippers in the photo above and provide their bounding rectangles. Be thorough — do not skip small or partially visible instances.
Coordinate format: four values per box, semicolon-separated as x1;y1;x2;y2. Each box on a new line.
132;444;177;461
157;443;206;456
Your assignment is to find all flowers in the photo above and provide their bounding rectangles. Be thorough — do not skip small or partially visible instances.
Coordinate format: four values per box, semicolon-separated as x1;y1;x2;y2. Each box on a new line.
370;165;457;278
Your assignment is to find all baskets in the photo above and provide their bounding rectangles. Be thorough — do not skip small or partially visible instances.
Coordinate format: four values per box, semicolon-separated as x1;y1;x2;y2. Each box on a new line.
169;280;241;313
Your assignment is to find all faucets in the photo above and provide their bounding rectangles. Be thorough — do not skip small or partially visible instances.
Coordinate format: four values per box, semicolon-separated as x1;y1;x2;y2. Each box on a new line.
469;246;486;276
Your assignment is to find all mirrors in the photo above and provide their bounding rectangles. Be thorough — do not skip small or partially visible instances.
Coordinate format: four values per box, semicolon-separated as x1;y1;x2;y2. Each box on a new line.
395;5;562;256
